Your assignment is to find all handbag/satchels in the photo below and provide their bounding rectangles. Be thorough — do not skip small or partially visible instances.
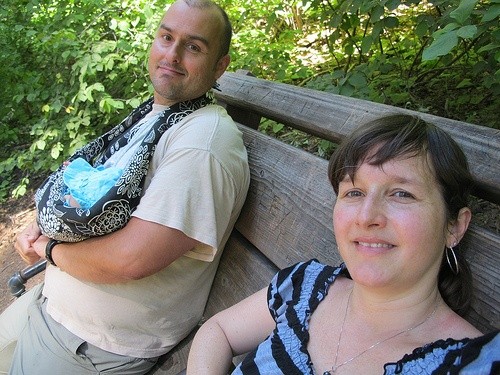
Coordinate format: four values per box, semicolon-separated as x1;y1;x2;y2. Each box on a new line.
35;94;210;242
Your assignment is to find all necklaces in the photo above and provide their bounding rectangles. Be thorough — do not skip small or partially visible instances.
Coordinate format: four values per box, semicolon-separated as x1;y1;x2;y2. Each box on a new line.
323;289;442;375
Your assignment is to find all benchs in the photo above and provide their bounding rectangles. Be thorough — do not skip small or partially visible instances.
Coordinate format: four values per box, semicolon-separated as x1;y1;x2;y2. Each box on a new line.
7;69;500;375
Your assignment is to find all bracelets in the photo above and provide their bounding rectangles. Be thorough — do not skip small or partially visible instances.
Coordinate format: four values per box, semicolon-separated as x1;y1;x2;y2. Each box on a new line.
45;239;62;267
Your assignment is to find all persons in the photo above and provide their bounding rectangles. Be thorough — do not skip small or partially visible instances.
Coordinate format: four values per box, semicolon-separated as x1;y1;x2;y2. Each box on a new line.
184;114;500;375
0;0;249;375
